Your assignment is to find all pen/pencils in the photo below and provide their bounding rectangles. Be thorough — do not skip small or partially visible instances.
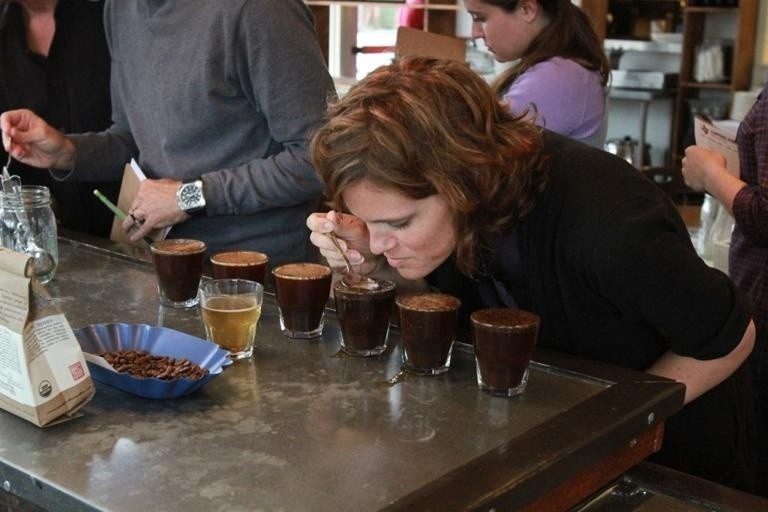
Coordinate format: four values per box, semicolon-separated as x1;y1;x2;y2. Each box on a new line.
93;188;155;245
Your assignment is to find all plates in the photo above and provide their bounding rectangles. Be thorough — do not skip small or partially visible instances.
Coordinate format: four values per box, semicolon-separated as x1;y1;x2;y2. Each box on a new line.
73;322;233;400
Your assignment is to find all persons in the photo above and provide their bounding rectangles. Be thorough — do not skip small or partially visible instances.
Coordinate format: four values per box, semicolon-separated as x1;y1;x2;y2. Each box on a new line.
460;0;608;151
1;0;122;238
304;58;765;499
683;79;766;333
0;2;342;290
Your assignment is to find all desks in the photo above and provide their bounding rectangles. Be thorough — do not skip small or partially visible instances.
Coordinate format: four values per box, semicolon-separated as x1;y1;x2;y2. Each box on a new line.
0;226;686;512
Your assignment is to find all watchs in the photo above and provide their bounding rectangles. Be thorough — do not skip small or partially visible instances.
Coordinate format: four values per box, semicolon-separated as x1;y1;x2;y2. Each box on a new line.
175;176;211;220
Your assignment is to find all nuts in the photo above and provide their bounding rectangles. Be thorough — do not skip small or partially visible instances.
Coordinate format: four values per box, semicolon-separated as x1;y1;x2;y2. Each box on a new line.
95;349;212;381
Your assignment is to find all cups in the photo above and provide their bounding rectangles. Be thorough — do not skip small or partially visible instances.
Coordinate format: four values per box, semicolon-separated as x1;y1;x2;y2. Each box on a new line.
396;291;461;377
198;250;269;359
271;262;332;339
469;308;541;398
333;277;396;358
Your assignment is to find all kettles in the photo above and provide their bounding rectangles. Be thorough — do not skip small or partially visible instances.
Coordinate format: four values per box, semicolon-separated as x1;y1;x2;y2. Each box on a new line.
604;136;653;173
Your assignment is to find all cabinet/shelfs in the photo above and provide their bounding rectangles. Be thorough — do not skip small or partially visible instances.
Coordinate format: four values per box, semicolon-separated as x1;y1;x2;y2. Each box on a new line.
413;0;760;211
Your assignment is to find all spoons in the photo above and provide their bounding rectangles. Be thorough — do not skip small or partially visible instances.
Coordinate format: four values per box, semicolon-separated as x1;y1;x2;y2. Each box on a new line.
148;238;207;309
0;173;56;276
325;229;380;290
